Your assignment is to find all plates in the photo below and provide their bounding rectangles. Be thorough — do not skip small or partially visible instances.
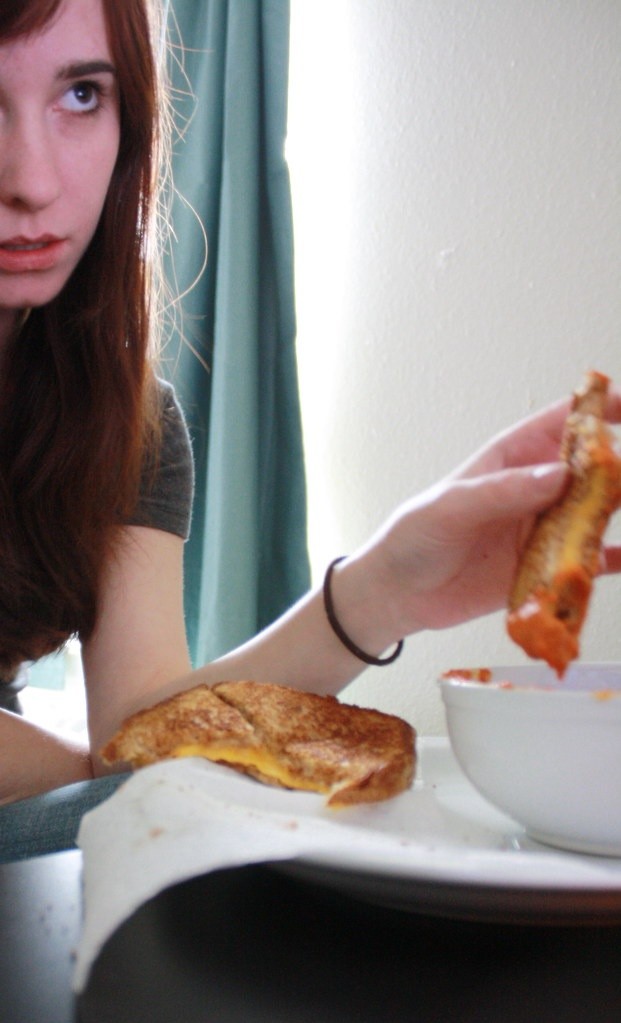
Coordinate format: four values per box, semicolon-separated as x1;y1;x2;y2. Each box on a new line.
72;736;621;999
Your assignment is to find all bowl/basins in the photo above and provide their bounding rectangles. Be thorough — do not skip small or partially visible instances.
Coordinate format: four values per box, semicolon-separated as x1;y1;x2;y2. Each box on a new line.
439;661;621;857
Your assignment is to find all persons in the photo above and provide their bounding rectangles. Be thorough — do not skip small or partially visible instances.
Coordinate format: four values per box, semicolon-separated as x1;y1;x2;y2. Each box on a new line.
0;0;621;862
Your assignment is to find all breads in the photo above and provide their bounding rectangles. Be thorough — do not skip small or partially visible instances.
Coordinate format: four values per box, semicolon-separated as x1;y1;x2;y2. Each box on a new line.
98;679;417;806
508;371;620;669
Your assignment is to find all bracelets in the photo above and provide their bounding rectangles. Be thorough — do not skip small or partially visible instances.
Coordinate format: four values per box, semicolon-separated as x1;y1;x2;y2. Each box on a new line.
322;555;403;666
